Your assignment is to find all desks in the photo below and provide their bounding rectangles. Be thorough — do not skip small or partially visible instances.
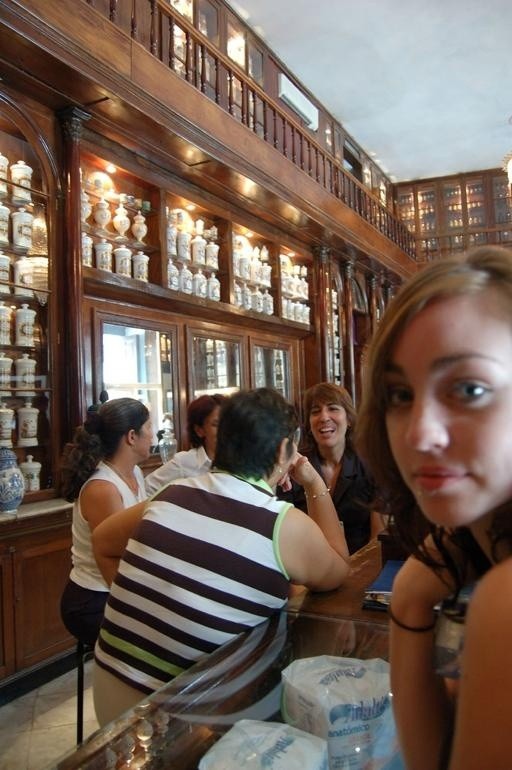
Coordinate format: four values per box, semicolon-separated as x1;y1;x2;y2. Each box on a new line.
287;521;434;621
57;614;462;770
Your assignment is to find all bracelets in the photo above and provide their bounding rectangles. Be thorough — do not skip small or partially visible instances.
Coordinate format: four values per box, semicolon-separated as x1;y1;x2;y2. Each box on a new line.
303;486;331;500
388;604;437;633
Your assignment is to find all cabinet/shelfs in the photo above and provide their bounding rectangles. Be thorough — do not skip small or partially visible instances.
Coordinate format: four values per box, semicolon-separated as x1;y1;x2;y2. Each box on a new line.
280;229;310;340
2;522;86;677
0;84;62;497
77;144;162;303
229;203;278;327
163;170;228;318
395;176;511;255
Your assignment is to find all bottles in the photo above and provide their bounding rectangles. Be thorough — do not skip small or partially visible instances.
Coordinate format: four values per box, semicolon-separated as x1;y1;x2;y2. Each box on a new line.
1;447;25;514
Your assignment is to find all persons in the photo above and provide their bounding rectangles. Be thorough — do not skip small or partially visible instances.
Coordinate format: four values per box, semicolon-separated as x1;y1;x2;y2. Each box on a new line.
142;393;231;498
351;244;511;769
52;397;155;651
90;386;352;732
276;380;384;556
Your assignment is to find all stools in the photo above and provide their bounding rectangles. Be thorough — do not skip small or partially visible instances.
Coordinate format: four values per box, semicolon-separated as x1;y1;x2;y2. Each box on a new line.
57;629;101;746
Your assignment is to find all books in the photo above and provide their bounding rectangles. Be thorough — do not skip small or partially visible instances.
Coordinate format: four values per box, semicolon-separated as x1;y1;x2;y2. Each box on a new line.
361;558;472;619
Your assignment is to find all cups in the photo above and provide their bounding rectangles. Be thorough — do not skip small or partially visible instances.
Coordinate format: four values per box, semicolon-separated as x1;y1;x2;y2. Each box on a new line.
1;151;48;494
324;286;343;387
157;429;177;465
78;230;151;284
164;207;310;327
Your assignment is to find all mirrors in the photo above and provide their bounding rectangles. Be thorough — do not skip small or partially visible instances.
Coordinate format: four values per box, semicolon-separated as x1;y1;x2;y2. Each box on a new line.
249;333;294;415
182;323;248;441
88;307;179;465
